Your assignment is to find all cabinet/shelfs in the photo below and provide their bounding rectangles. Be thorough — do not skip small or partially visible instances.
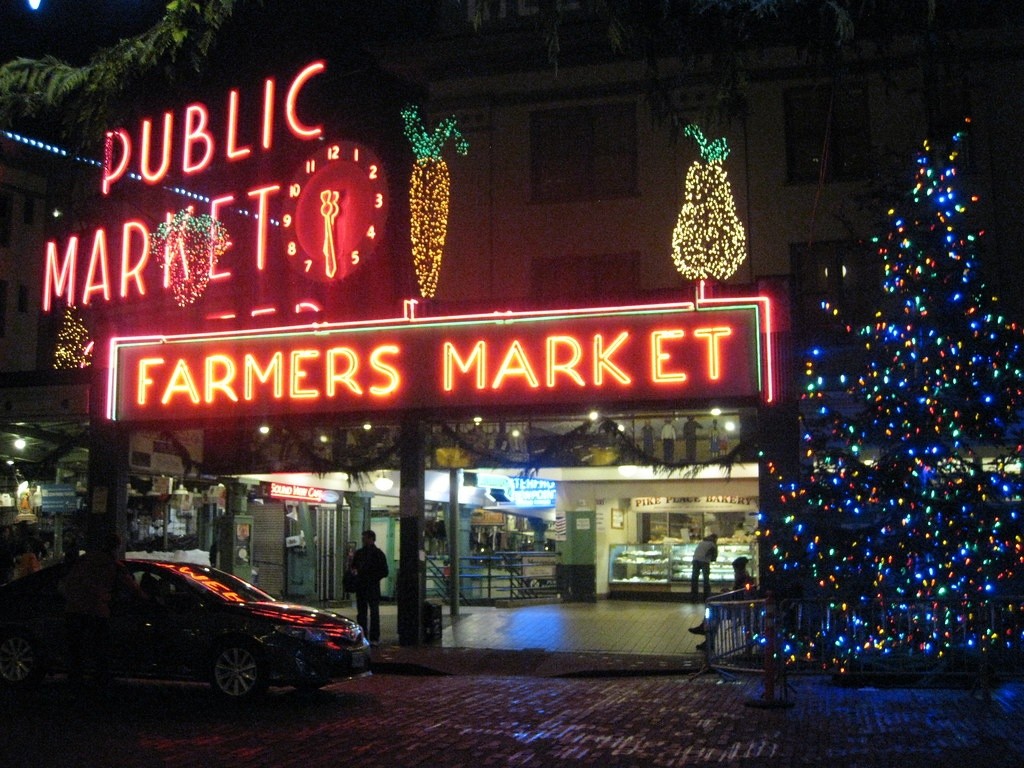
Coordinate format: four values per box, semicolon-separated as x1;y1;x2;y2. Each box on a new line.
608;543;755;600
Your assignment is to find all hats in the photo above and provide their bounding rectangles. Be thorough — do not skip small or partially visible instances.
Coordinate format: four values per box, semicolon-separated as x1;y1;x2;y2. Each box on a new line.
732;556;749;567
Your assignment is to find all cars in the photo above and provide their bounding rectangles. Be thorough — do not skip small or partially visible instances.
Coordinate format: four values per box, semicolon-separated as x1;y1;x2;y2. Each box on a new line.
0;557;376;712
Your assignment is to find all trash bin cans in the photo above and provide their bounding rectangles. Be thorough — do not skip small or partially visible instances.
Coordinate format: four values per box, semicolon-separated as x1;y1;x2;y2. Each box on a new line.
425;603;443;642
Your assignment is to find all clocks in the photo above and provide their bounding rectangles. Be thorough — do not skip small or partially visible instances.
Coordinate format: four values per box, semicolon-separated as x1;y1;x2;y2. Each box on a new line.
278;140;390;283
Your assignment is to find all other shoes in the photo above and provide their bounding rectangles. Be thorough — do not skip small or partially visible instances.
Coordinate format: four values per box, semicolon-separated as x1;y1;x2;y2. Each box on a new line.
371;640;379;646
688;626;705;635
692;598;698;603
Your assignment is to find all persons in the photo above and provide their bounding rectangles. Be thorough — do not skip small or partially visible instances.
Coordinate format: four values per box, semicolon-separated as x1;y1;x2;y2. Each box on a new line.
0;521;50;585
690;534;718;602
349;531;389;646
64;533;148;707
689;557;751;650
